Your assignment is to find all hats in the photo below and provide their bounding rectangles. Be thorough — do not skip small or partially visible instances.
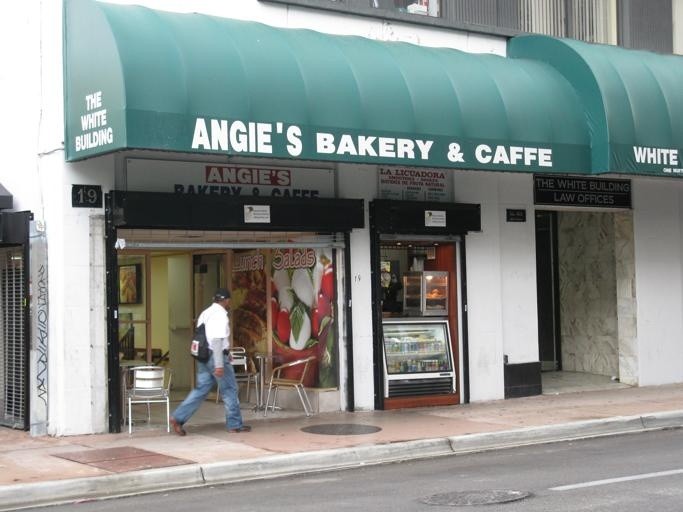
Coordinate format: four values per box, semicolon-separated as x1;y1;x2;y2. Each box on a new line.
214;289;231;301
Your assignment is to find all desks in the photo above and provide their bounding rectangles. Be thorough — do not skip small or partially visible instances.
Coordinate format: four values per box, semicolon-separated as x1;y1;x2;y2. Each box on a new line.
119;359;155;426
245;352;282;412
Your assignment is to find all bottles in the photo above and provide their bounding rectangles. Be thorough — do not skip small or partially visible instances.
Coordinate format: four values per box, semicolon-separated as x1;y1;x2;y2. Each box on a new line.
384;339;447;372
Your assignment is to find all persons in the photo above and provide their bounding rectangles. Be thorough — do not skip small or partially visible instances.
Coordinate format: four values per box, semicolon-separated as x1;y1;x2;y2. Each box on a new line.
167;288;251;435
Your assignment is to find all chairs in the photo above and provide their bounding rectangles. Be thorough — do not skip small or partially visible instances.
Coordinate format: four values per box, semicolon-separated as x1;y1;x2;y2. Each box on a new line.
264;356;317;417
216;346;260;408
124;365;173;435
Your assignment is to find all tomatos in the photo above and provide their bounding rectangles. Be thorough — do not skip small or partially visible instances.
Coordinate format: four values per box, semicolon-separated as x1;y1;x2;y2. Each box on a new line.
271;264;333;350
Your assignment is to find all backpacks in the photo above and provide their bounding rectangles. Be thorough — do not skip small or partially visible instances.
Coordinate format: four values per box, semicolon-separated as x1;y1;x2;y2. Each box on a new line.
189;323;213;364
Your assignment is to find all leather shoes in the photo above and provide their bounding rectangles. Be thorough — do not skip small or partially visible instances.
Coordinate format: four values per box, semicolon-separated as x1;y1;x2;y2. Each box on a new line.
169;416;187;436
227;426;252;433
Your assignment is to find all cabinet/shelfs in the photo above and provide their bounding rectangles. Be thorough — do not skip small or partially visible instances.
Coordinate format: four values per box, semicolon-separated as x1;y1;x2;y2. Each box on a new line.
380;317;460;410
402;269;449;317
